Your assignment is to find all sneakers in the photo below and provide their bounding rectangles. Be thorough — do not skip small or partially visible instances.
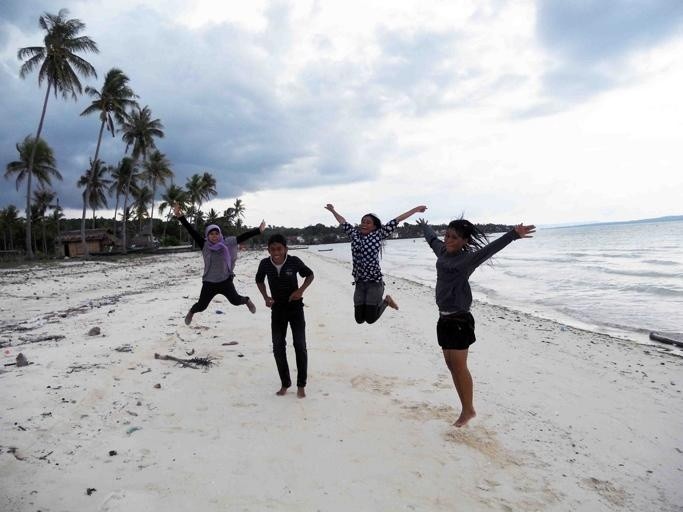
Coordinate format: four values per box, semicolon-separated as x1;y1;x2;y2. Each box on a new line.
185;308;193;324
246;296;256;314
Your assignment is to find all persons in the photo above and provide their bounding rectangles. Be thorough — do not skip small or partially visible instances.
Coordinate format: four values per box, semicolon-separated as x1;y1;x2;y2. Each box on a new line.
256;235;314;399
171;199;266;326
324;202;429;323
414;216;536;428
